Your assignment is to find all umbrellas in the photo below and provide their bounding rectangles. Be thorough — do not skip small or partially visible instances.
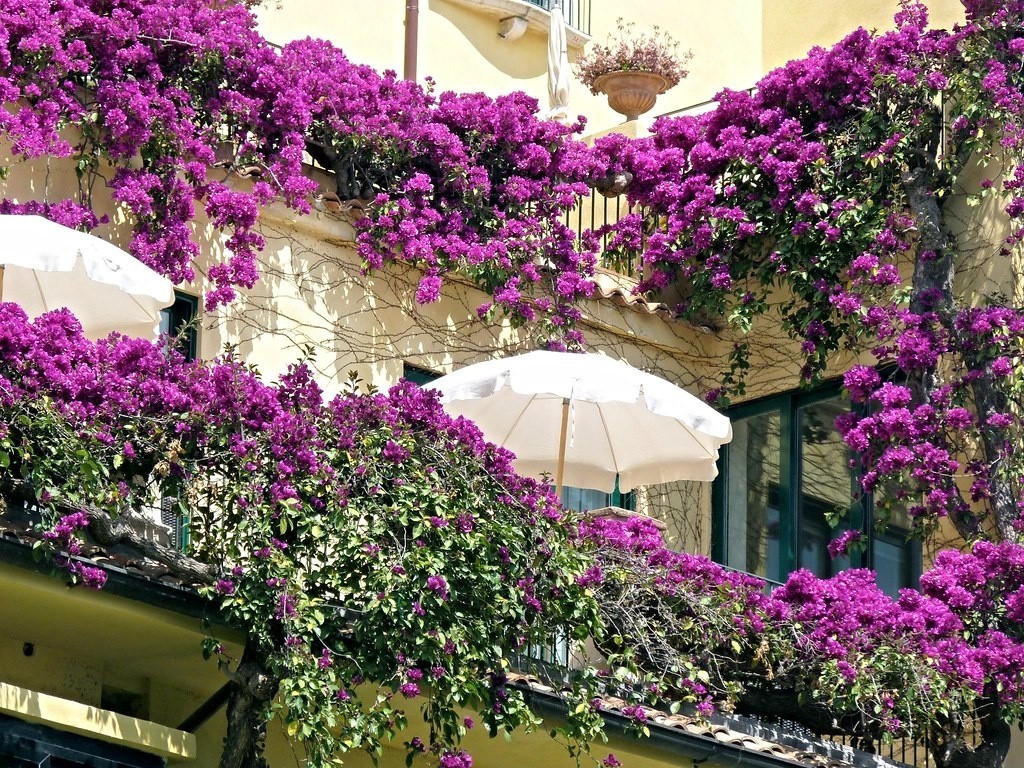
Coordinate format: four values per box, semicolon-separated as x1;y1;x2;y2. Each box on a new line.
2;213;177;346
415;349;734;513
